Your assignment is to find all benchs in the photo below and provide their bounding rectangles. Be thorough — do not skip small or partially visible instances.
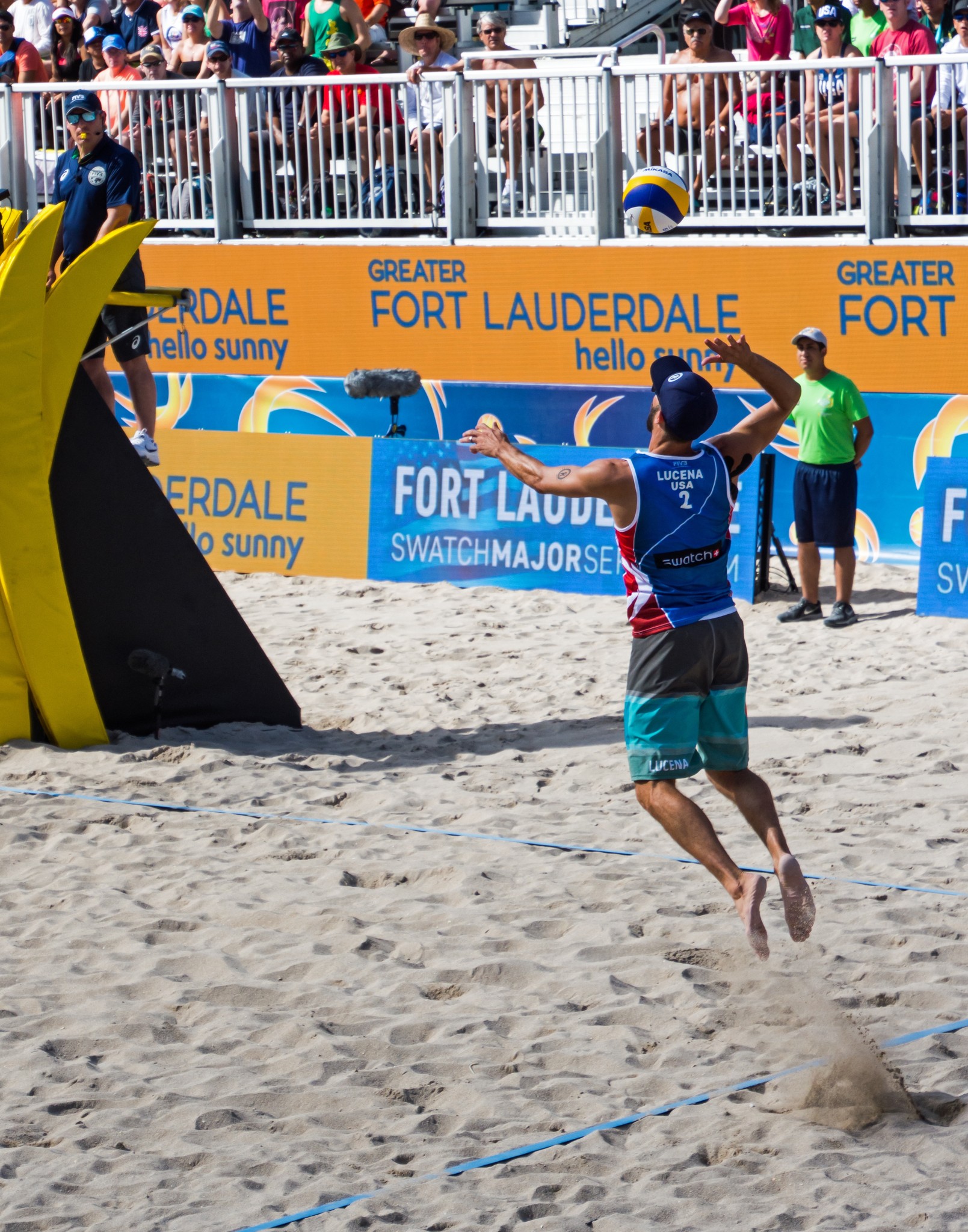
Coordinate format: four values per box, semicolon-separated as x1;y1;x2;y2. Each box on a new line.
679;142;966;191
35;7;506;173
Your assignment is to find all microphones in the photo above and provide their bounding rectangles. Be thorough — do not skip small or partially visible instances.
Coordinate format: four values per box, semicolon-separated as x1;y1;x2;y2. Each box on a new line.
96;129;103;135
343;369;422;399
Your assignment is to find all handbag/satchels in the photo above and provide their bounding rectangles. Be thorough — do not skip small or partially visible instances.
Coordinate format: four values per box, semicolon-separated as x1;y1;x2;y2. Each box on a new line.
756;176;821;237
740;99;799;148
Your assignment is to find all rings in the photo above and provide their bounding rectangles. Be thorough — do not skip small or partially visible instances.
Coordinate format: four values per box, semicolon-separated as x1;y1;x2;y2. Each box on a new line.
468;436;473;444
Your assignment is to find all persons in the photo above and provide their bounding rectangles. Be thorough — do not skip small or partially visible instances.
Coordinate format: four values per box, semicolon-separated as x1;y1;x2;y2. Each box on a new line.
44;92;158;469
457;335;816;961
777;333;875;628
0;0;547;220
635;0;968;238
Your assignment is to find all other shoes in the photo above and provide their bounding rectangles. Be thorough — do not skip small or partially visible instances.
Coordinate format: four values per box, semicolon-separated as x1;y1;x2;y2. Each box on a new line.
806;155;817;171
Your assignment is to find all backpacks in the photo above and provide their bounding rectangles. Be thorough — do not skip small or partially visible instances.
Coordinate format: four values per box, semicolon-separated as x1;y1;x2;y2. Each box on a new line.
138;172;216;238
914;165;967;215
288;165;479;238
0;37;26;84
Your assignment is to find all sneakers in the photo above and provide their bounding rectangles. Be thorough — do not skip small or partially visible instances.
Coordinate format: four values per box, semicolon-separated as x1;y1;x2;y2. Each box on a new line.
777;596;823;623
825;601;858;628
493;193;520;213
128;427;161;467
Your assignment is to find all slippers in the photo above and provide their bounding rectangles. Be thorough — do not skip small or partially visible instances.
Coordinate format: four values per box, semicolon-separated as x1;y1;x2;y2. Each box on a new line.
370;57;399;66
821;198;856;212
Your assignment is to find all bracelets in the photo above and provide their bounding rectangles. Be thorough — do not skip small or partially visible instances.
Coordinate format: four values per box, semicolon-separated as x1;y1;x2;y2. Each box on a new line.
105;129;114;139
652;119;659;123
144;124;150;135
298;122;305;129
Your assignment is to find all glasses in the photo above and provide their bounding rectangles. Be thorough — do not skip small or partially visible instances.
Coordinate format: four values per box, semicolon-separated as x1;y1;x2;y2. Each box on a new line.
953;14;968;21
686;28;706;35
816;18;843;28
53;18;505;68
0;24;13;31
65;110;103;125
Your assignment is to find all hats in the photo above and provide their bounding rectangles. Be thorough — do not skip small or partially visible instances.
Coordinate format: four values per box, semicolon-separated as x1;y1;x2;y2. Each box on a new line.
64;89;102;119
791;327;827;348
53;4;456;63
684;9;712;24
650;355;718;437
813;5;844;23
952;0;968;15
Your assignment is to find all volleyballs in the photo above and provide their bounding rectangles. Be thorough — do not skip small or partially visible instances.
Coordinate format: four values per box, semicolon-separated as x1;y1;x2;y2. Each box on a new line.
622;165;690;234
